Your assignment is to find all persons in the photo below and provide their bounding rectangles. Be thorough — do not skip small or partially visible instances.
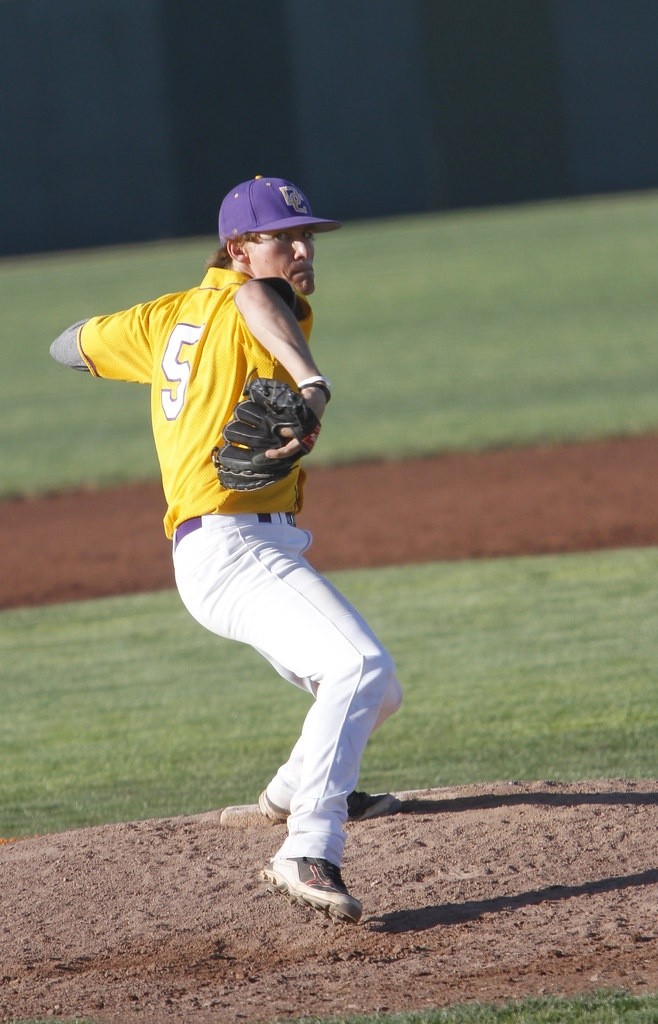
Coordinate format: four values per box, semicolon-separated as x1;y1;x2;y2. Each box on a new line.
49;174;404;924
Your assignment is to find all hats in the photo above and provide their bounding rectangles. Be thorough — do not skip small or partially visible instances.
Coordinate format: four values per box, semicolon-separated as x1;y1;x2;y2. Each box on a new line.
219;173;341;247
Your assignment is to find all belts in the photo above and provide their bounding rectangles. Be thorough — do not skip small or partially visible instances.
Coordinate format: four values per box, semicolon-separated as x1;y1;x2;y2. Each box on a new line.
176;513;296;545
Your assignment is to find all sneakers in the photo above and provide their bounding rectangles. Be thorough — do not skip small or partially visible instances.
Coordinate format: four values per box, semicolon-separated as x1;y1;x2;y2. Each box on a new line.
260;859;361;922
260;789;290;822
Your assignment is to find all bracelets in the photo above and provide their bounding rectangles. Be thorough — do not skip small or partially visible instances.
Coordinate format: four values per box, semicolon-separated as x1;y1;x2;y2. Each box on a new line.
298;376;331;388
299;384;330;404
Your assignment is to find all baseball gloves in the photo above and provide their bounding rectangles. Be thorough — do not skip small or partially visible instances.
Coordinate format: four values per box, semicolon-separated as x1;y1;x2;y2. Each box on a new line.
217;378;322;490
